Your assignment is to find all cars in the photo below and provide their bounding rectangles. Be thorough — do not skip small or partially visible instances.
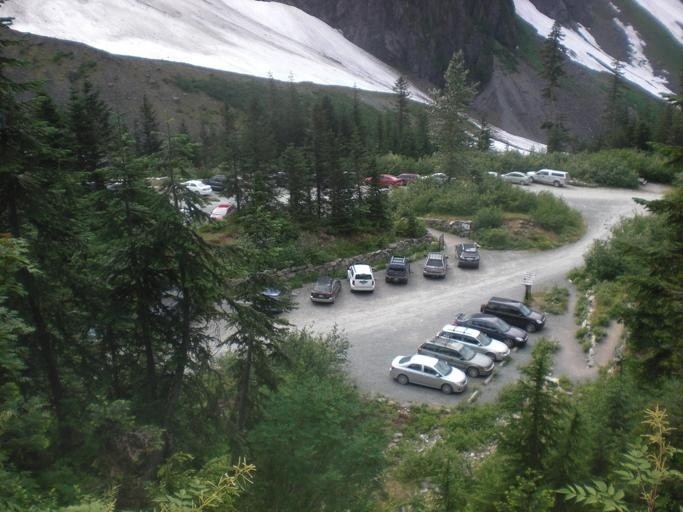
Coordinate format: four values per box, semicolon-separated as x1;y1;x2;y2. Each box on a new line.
388;296;548;396
397;173;422;185
500;171;533;186
365;173;405;186
175;175;240;227
310;276;343;305
471;171;500;181
421;172;457;184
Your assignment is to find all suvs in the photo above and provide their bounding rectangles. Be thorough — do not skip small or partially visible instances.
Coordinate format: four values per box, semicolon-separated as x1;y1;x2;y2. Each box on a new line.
422;252;451;279
347;264;378;294
454;242;482;271
385;254;413;287
257;286;294;314
527;168;571;187
